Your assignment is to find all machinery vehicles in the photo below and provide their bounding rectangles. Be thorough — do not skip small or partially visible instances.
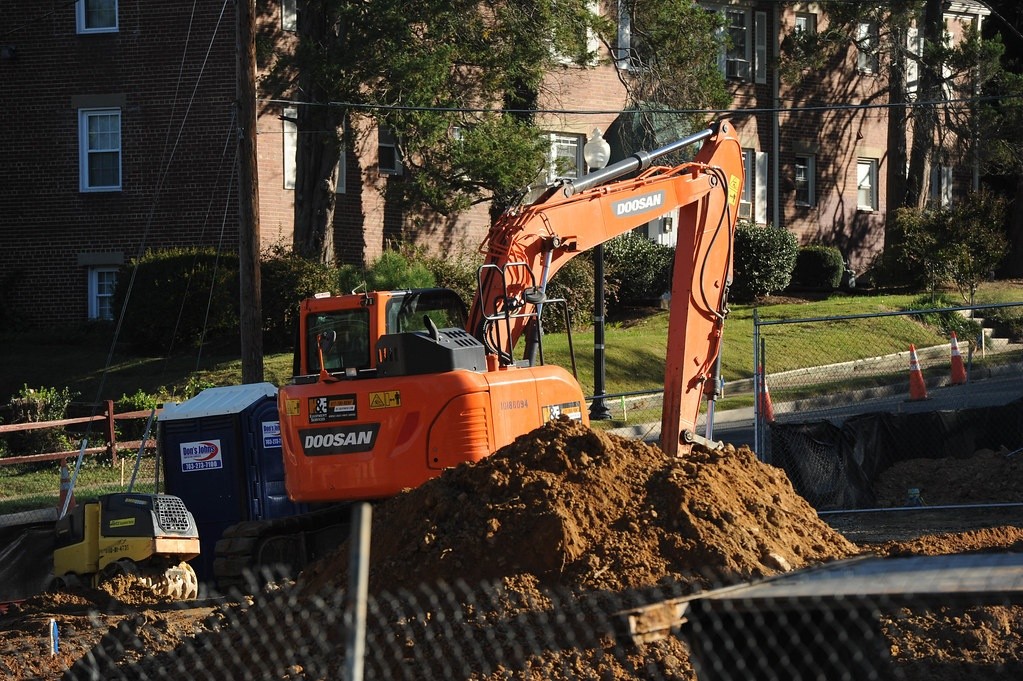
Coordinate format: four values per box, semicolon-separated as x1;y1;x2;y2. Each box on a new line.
278;112;748;523
46;483;205;606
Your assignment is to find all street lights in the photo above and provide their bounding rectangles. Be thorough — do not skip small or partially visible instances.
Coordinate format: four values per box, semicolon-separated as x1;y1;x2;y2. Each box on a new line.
583;128;612;422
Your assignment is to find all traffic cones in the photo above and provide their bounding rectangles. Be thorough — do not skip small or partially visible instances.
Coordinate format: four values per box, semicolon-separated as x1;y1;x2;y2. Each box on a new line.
56;459;80;511
948;330;970;385
751;364;775;424
904;343;931;402
